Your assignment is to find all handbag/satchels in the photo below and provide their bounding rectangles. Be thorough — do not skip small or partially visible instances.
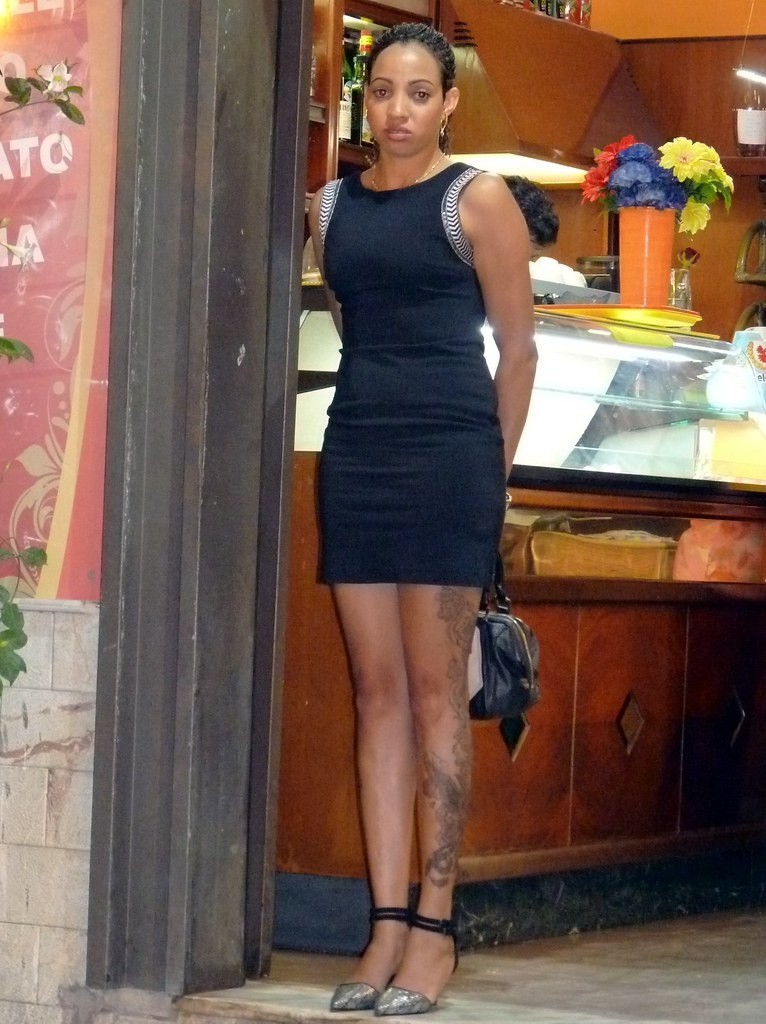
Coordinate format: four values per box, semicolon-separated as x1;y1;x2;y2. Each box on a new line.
467;548;540;719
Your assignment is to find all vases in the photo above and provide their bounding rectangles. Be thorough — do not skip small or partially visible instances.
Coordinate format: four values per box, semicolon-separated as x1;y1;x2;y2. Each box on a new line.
668;268;693;310
616;206;678;306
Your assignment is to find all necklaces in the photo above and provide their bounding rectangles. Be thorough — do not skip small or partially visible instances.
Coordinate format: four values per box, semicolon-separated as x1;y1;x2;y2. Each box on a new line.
372;153;447;192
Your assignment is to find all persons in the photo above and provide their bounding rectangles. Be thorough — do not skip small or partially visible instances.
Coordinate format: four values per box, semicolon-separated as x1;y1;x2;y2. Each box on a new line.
503;174;557;261
309;22;537;1018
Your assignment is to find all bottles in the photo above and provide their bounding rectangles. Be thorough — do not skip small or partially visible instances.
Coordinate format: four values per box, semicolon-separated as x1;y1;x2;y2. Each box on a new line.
494;0;592;29
339;29;374;149
734;81;766;157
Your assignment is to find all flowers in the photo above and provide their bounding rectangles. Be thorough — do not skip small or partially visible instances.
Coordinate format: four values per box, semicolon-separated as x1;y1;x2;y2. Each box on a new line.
676;248;700;270
580;135;735;242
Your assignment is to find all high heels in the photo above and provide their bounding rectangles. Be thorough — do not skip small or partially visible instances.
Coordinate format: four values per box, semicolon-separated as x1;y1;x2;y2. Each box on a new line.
331;909;413;1010
375;912;459;1015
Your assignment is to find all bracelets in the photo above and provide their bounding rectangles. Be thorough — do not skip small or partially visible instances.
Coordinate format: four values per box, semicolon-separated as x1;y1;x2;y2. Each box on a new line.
505;492;513;510
